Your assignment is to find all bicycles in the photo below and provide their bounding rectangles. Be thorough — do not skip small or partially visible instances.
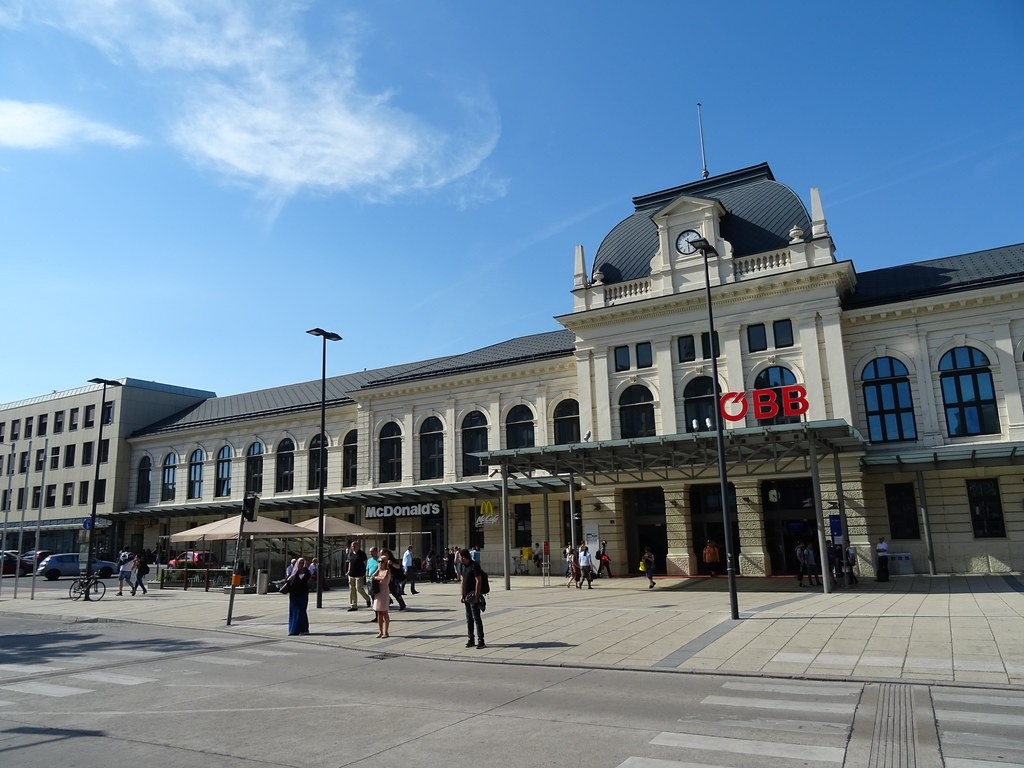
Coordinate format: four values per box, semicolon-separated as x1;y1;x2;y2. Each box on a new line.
68;570;107;602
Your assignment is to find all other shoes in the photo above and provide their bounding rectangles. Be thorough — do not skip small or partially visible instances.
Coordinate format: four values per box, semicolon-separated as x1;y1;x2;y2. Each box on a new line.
143;590;147;594
130;591;132;593
566;583;570;588
476;642;485;649
132;591;136;596
609;575;614;579
576;586;579;588
649;585;653;589
579;583;582;589
347;588;420;623
382;634;389;638
588;587;593;589
375;634;382;638
115;592;123;596
653;582;656;586
465;640;475;648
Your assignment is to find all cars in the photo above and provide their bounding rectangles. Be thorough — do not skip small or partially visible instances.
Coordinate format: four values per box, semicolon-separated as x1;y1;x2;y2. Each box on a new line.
6;550;55;565
0;550;35;577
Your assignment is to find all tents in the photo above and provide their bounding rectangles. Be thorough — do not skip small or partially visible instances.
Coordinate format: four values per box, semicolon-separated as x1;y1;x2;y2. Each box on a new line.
163;513;320;590
263;514;386;580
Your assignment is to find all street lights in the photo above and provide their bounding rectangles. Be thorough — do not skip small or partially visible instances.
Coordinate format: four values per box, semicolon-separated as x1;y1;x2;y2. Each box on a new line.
307;326;343;611
83;377;124;600
687;236;744;621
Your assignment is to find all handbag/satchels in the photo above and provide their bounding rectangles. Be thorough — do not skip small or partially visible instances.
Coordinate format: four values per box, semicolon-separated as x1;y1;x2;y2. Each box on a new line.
638;561;647;571
590;570;597;579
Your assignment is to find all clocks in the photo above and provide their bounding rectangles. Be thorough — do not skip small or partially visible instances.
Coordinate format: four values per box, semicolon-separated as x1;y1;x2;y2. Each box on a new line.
675;228;701;255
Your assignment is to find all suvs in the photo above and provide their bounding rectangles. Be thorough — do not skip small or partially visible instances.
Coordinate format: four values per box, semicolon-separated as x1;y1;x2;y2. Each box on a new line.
167;550;218;569
36;553;120;580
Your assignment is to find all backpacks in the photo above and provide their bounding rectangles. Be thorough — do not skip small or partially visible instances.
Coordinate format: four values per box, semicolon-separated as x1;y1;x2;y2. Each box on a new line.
141;565;150;575
481;571;490;594
563;549;567;558
595;550;601;560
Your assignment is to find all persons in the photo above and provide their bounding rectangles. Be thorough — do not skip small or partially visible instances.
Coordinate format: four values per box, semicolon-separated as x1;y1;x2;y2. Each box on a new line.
425;546;483;583
532;543;545;576
371;554;392;638
400;545;420;595
286;558;330;591
458;548;486;649
793;539;860;587
285;558;311;635
115;544;135;596
876;536;889;577
561;538;595;589
130;553;148;594
702;539;722;578
598;540;615;578
641;547;656;588
345;541;371;612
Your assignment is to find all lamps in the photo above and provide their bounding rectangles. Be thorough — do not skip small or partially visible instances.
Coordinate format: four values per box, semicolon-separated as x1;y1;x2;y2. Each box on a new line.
826;501;838;509
488;467;502;477
692;418;698;430
583;430;590;442
705;417;712;430
593;503;600;510
742;497;750;503
669;500;677;506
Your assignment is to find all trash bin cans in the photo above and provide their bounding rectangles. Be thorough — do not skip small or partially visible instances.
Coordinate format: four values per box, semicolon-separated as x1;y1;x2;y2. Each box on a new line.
256;568;269;594
